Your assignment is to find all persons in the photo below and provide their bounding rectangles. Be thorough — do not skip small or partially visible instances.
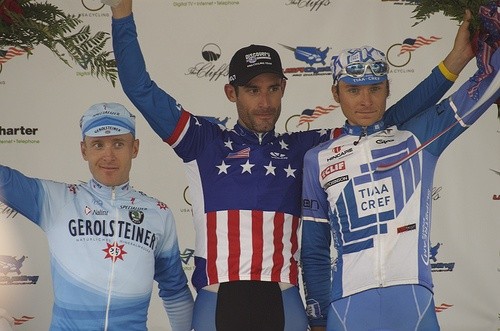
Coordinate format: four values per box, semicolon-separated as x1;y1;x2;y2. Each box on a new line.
298;45;500;331
0;102;194;331
101;1;477;331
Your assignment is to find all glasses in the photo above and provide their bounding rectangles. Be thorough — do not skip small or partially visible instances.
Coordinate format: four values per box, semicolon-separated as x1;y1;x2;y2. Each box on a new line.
334;61;389;79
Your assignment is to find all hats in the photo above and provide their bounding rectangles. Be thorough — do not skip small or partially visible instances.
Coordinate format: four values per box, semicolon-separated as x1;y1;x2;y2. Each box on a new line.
333;46;391;85
79;103;136;138
228;44;288;85
